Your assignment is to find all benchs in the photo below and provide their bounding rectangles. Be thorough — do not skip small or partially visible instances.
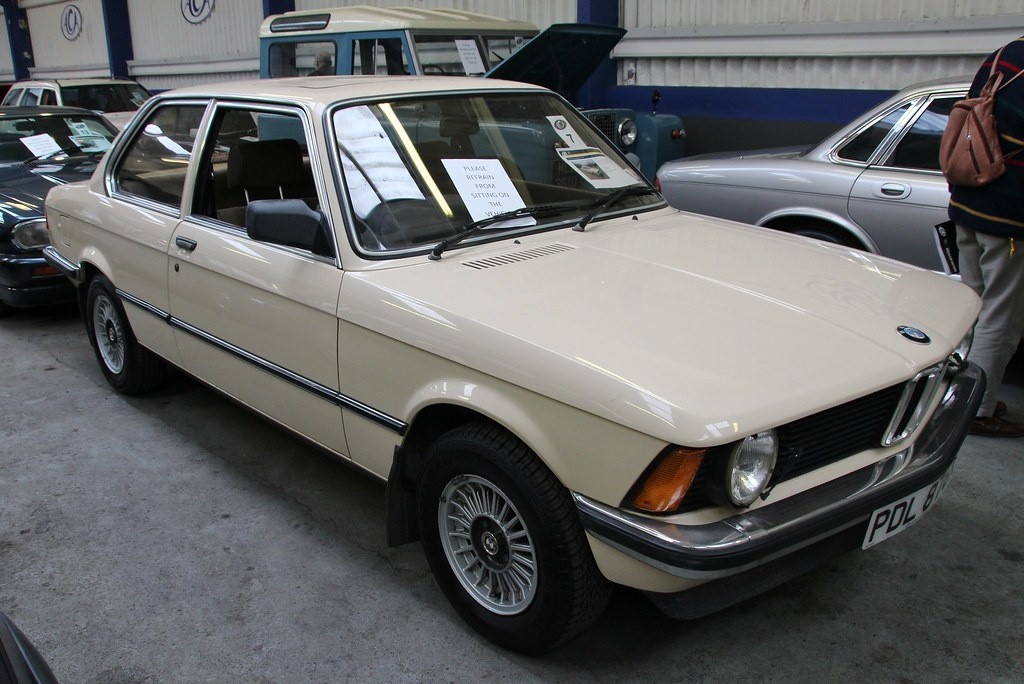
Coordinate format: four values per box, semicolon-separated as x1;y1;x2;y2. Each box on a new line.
122;159;319;214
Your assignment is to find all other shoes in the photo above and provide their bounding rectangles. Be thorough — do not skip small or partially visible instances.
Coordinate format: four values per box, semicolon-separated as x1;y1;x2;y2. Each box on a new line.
968;401;1024;437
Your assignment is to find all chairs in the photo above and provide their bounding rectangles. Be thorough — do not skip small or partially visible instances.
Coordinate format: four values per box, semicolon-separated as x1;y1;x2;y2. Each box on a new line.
403;141;470;196
224;138;305;238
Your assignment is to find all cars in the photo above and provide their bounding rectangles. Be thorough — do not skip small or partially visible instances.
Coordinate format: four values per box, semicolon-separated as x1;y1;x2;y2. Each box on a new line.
0;106;120;317
656;75;987;282
42;75;987;655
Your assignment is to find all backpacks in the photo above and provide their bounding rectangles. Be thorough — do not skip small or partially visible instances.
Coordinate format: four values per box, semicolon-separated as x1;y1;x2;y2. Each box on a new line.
939;45;1024;187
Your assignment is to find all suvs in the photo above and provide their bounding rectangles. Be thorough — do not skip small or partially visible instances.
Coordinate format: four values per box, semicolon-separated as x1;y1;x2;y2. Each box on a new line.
1;78;153;131
260;5;686;192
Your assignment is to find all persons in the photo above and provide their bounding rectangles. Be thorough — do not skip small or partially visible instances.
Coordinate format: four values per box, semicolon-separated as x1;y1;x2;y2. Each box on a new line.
306;51;333;77
948;36;1024;439
